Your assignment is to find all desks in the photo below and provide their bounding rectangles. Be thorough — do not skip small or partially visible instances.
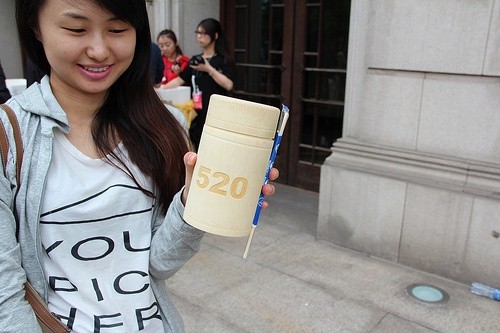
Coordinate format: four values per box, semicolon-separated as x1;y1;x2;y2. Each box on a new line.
151;85;191;131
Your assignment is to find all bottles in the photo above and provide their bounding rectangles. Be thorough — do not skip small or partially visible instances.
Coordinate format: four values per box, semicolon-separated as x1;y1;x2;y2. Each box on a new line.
470;281;500;301
182;94;280;237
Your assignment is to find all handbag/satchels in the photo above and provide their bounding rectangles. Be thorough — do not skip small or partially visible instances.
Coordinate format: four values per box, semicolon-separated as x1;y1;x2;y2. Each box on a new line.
1;103;72;332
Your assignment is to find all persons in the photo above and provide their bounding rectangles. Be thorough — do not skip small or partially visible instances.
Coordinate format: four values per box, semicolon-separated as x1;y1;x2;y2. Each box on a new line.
0;0;280;333
151;18;238;158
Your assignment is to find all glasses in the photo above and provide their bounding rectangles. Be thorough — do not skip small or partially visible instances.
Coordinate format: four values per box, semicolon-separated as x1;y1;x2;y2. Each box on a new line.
195;30;207;35
